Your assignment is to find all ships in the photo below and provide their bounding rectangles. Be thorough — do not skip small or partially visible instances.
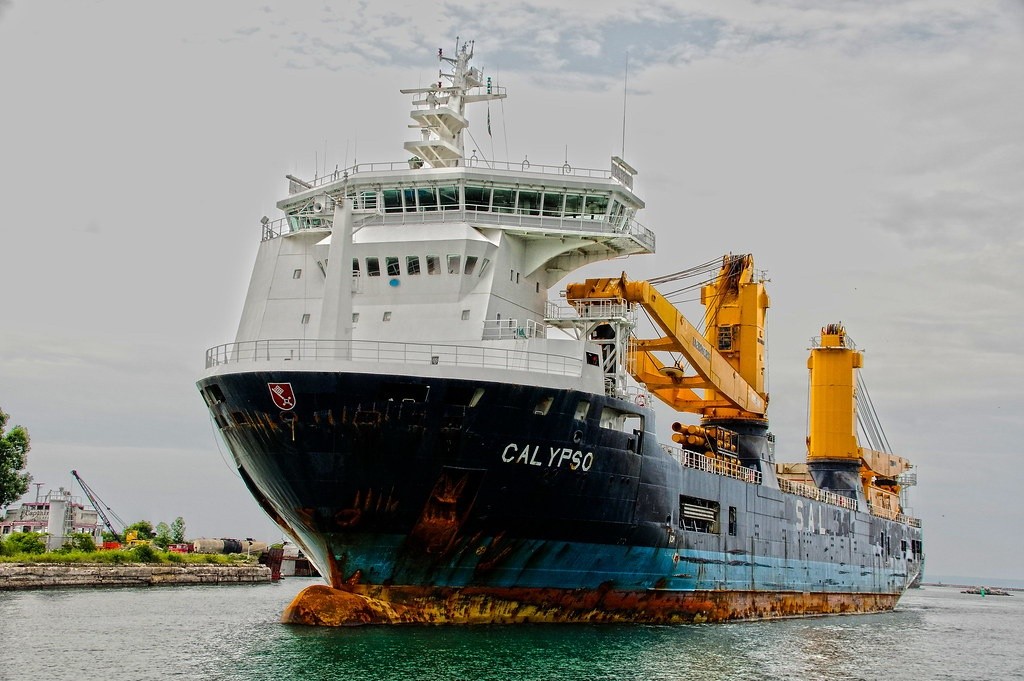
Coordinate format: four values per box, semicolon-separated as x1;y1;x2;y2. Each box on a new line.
195;33;925;630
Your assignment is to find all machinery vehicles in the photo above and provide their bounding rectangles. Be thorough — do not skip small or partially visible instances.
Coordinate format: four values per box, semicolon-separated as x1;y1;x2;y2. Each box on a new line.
70;469;155;551
